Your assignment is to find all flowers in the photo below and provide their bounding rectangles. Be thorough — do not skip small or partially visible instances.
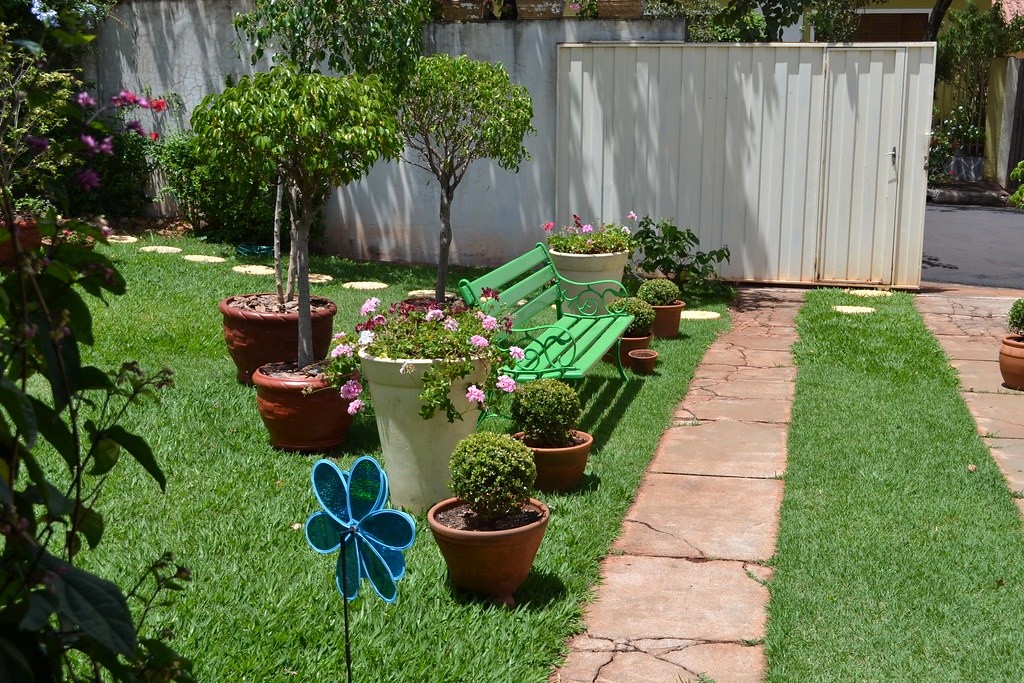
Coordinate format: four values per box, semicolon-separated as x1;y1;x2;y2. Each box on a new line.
540;211;637;252
330;288;524;422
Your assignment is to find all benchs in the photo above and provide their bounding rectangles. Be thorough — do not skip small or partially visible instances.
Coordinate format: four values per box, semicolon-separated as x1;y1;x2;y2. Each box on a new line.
459;243;635;428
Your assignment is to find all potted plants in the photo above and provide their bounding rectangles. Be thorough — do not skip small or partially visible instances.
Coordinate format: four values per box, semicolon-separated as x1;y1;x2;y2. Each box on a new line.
191;65;402;452
218;0;433;383
511;378;593;490
428;432;549;593
400;54;538;314
601;297;656;366
638;279;685;338
999;299;1024;391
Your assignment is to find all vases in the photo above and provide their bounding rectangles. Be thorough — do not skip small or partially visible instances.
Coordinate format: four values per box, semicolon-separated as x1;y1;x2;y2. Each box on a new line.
358;346;492;514
549;248;629;315
628;349;659;374
